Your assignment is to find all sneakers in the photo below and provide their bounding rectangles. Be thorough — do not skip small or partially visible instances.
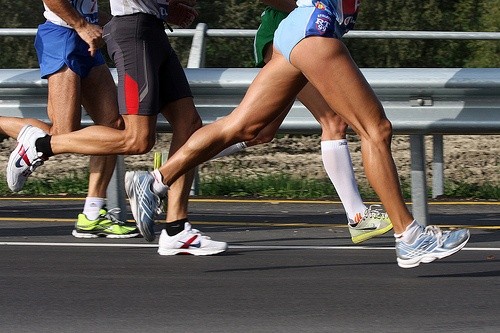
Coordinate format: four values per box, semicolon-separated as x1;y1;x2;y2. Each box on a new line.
392;221;471;271
4;123;49;192
125;168;162;244
156;223;228;259
345;208;395;243
71;207;143;240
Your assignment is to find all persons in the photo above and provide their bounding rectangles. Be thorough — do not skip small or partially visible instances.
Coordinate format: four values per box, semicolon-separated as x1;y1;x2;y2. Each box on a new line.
6;1;228;257
124;0;470;270
1;1;139;239
151;0;394;245
